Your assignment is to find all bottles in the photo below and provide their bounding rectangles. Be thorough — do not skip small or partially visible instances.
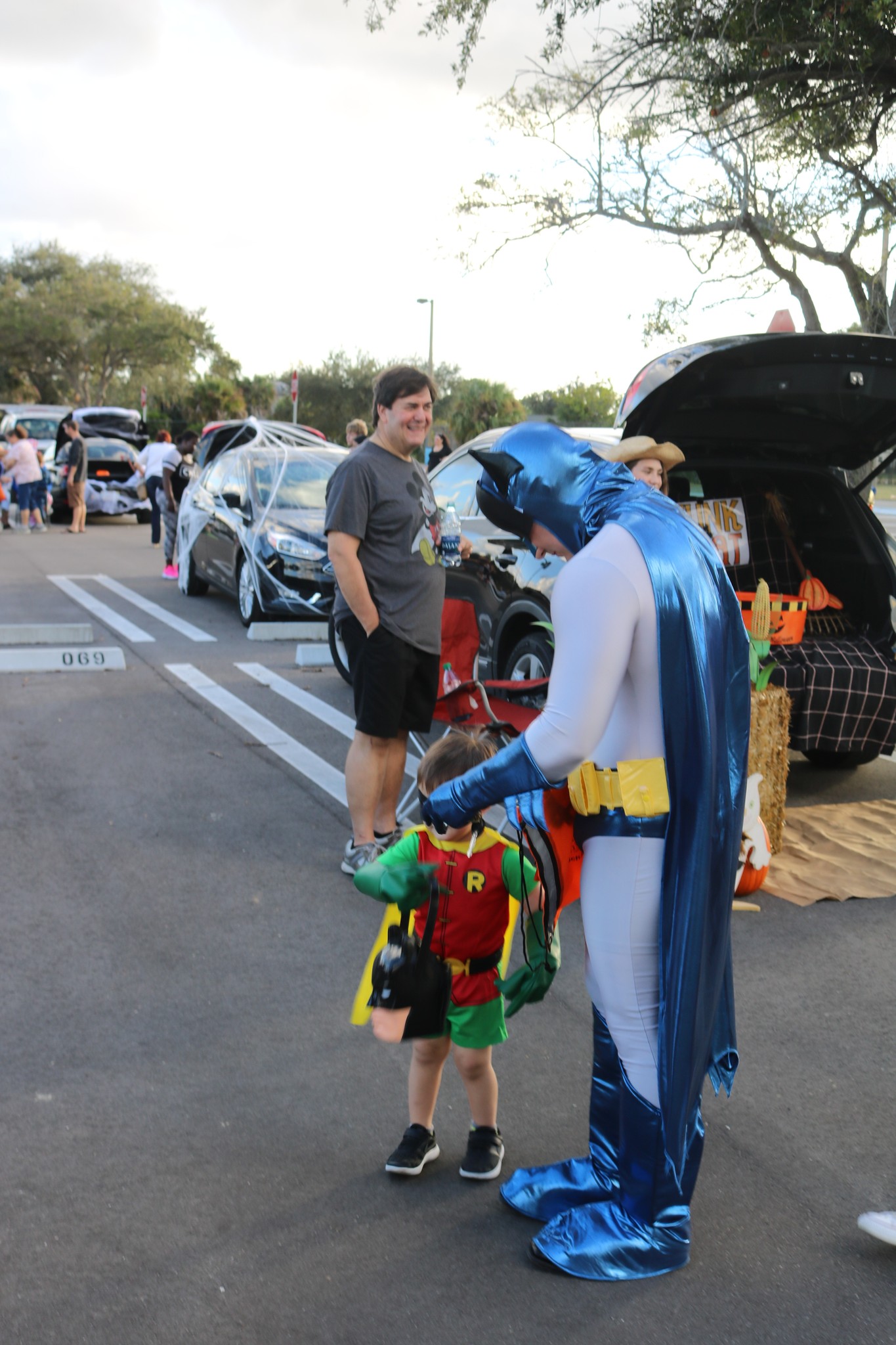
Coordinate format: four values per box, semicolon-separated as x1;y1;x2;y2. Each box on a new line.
379;924;402;1000
441;501;463;567
442;662;460;696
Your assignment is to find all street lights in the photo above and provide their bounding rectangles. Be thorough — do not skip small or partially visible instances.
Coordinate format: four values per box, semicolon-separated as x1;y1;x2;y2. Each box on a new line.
416;298;435;456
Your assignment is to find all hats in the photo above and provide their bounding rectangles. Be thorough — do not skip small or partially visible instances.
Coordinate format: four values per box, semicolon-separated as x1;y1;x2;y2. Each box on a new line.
592;436;686;471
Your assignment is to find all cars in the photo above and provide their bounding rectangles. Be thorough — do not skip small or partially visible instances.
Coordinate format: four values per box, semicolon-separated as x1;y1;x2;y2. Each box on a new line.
0;403;154;525
175;418;352;630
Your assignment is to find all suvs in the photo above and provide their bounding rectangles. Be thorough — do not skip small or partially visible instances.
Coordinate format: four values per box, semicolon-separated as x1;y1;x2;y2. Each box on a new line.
327;331;896;771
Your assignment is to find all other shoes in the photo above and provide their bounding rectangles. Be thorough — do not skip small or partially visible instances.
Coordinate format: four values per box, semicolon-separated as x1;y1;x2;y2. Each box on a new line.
67;526;87;534
14;527;31;535
31;523;47;533
3;523;11;529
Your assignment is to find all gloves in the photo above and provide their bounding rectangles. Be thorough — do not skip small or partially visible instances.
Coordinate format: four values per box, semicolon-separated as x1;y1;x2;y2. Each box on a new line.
494;910;564;1020
352;861;442;909
420;729;564;835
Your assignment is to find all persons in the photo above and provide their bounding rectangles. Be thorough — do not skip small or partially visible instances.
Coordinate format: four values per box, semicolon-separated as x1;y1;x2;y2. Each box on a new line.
857;1211;896;1246
138;430;177;548
156;430;200;580
353;732;558;1180
610;436;685;490
346;418;368;451
323;367;473;875
60;422;87;534
0;425;46;533
420;418;750;1283
426;434;452;474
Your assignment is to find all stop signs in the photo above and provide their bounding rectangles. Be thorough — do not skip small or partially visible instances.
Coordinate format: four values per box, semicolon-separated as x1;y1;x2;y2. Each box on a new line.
290;371;299;402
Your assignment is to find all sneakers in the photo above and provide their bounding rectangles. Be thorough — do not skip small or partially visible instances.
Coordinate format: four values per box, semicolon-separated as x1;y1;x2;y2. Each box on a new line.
339;835;388;875
371;826;403;849
162;566;180;579
386;1121;441;1175
459;1124;506;1178
